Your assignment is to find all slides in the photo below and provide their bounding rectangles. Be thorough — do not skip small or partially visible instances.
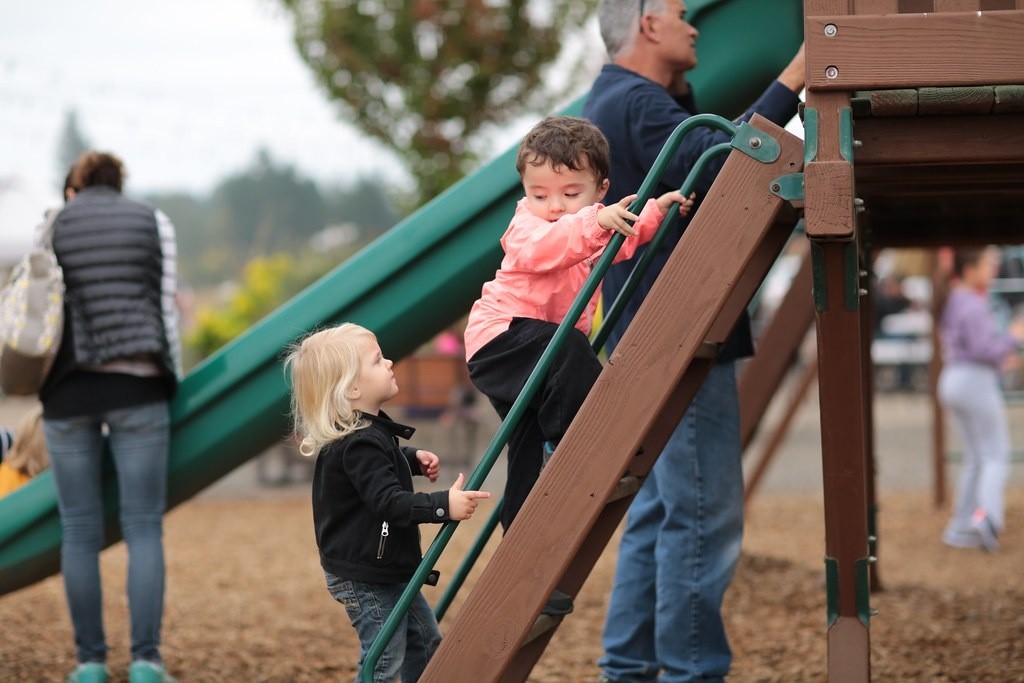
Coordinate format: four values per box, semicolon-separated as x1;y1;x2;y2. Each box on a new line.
0;1;804;613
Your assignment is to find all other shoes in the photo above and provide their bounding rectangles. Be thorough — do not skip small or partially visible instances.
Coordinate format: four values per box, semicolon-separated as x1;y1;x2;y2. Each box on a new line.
68;662;178;682
539;593;574;615
940;506;1003;556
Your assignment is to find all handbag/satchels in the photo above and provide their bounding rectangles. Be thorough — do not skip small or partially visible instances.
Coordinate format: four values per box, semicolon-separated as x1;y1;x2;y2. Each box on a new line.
1;207;67;400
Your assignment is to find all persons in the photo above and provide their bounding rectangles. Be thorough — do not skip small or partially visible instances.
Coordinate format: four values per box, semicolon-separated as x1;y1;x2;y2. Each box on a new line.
934;241;1018;553
461;117;691;616
584;1;804;683
34;151;182;683
0;410;51;498
283;320;491;683
